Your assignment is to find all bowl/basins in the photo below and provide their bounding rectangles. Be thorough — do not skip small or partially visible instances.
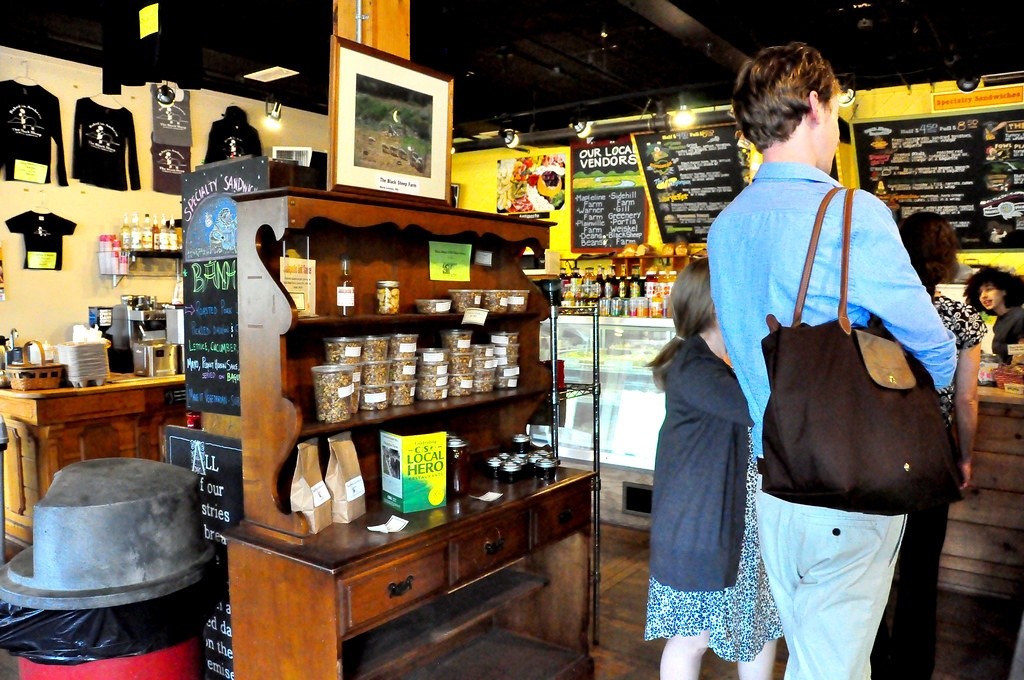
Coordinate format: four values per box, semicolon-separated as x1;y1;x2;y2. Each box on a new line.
414;298;452;314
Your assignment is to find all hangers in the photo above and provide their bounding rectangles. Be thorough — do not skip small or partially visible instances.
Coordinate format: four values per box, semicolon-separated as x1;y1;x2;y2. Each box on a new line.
89;92;123;108
13;60;37;84
34;191;50;213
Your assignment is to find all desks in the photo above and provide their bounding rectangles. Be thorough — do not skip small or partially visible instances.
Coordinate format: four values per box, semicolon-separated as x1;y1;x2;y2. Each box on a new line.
222;469;596;680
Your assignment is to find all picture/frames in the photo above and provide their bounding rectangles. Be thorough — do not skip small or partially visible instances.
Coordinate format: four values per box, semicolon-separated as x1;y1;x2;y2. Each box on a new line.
325;34;457;205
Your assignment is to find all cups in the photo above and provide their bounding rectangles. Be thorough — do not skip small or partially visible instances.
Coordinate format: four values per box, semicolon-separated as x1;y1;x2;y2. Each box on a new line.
507;289;529;314
8;347;21;363
415;328;521;400
322;333;419;413
481;290;510;313
310;365;356;423
446;288;483;314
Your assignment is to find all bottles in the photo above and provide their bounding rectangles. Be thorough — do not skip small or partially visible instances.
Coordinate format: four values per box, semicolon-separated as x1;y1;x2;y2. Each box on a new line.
159;213;182;250
121;294;158;311
97;234;130;274
119;213;131;250
512;433;531;454
141;213;154;251
375;280;400;315
336;256;357;317
644;270;677;298
150;215;161;250
131;212;141;250
447;436;469;497
487;450;557;483
559;264;643;300
0;335;9;370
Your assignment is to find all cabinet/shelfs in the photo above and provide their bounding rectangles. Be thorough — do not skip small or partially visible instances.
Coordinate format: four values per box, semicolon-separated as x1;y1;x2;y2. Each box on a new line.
232;184;566;534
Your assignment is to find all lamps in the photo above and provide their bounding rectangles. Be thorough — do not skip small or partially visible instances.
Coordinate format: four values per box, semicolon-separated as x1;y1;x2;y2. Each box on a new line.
265;102;282;121
498;128;519;148
949;57;981;92
156;81;174;107
572;120;592;138
674;96;694;127
839;76;856;108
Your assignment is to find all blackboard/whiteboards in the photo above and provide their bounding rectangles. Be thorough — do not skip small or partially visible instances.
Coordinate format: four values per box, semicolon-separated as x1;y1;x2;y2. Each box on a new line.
180;259;242;417
848;103;1024;254
567;140;650;253
164;425;245;680
628;122;843;245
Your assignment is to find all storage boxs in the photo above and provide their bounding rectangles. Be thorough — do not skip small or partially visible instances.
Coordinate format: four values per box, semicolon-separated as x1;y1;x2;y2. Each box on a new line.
380;429;448;513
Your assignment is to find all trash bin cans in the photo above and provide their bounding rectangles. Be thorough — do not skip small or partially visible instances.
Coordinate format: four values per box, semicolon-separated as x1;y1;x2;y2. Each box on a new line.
0;456;217;680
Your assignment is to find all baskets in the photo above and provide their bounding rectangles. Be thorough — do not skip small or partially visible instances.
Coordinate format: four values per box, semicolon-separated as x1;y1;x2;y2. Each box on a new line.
3;340;64;391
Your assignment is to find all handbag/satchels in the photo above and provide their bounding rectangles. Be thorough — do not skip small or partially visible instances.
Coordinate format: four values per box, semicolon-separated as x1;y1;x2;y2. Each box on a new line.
761;189;965;515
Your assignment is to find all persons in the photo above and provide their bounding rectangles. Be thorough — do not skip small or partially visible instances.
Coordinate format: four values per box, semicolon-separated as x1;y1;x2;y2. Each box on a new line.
706;43;958;680
870;212;988;680
963;266;1024;366
645;255;784;680
